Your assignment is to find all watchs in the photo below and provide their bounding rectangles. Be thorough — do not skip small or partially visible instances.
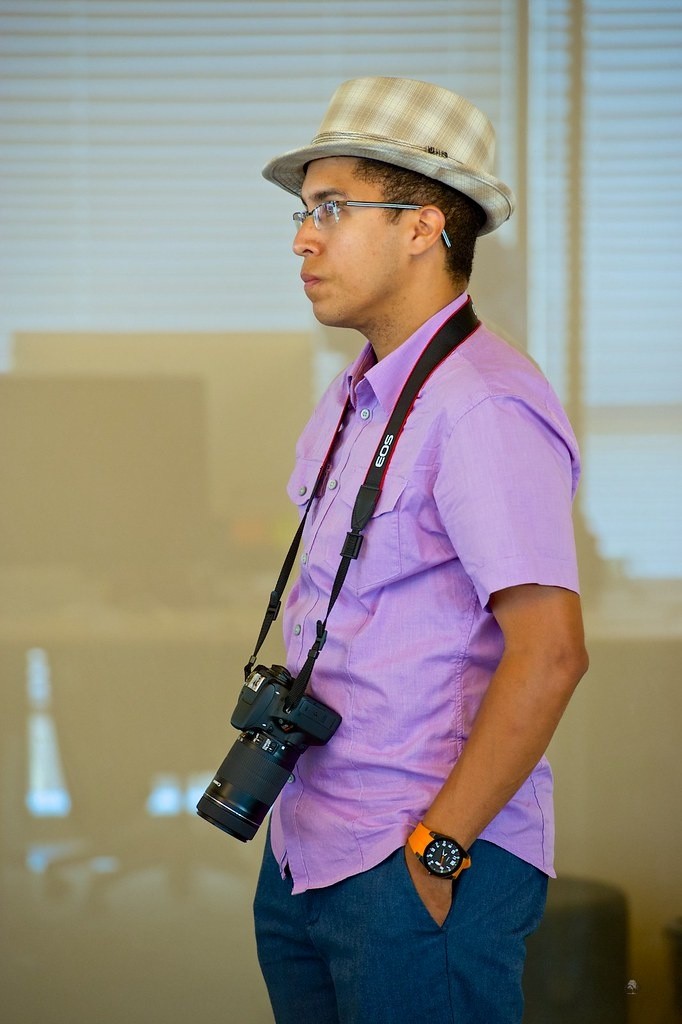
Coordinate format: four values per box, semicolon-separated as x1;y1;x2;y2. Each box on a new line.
407;821;471;880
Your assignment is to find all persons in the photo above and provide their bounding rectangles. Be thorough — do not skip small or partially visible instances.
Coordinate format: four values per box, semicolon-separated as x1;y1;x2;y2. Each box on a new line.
196;77;589;1024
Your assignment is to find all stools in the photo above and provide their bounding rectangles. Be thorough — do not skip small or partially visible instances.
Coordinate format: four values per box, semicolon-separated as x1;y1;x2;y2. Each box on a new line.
522;873;629;1024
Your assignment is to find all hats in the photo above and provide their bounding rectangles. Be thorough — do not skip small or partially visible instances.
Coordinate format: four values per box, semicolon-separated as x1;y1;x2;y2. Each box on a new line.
261;77;516;236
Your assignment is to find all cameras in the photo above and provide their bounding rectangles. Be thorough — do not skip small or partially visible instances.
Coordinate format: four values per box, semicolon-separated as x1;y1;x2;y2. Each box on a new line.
196;664;342;843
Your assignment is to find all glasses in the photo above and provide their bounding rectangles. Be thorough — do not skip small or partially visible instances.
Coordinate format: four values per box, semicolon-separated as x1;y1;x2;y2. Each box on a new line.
291;201;453;249
